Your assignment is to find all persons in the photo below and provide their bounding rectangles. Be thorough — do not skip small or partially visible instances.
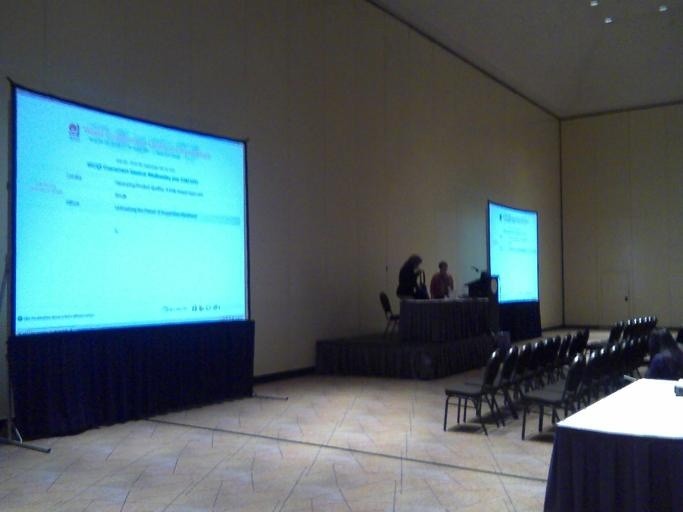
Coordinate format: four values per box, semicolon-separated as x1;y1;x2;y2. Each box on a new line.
429;261;453;299
622;326;682;387
396;255;423;300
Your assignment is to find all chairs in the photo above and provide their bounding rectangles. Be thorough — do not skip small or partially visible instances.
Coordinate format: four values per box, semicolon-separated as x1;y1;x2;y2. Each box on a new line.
442;317;657;440
380;290;400;334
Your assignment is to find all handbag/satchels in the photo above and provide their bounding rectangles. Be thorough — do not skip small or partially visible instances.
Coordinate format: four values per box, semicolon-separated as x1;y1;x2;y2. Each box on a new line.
414;283;430;300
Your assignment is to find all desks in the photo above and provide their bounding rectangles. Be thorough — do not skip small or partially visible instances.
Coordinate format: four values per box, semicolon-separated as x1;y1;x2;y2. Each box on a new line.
405;297;493;340
545;378;683;512
